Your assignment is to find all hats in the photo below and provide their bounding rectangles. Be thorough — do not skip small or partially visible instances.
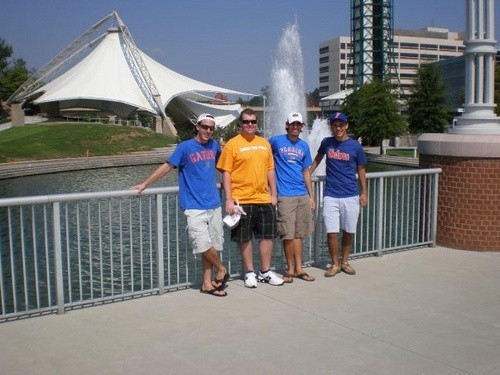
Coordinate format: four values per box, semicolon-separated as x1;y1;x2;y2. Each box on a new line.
286;112;303;124
329;112;347;124
196;113;215;124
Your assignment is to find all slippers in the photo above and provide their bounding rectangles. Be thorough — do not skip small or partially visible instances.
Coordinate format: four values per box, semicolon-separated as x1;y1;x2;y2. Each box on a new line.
212;273;228;289
294;273;315;281
200;287;228;297
282;273;295;282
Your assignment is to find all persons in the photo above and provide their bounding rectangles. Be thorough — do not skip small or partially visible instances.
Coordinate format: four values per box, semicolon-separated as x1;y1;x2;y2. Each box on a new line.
310;111;367;276
131;112;230;296
268;114;316;283
358;136;362;144
217;108;284;287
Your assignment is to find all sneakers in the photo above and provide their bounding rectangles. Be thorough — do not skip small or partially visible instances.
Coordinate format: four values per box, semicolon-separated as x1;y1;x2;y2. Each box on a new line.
257;269;285;285
245;272;258;288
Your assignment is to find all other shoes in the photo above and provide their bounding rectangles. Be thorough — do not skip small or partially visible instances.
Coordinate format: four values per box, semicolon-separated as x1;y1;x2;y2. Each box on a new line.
340;263;355;274
325;265;342;277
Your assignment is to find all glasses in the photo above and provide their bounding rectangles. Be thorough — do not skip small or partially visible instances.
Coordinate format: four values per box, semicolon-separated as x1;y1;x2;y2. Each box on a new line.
241;119;258;124
291;122;304;126
199;124;215;131
